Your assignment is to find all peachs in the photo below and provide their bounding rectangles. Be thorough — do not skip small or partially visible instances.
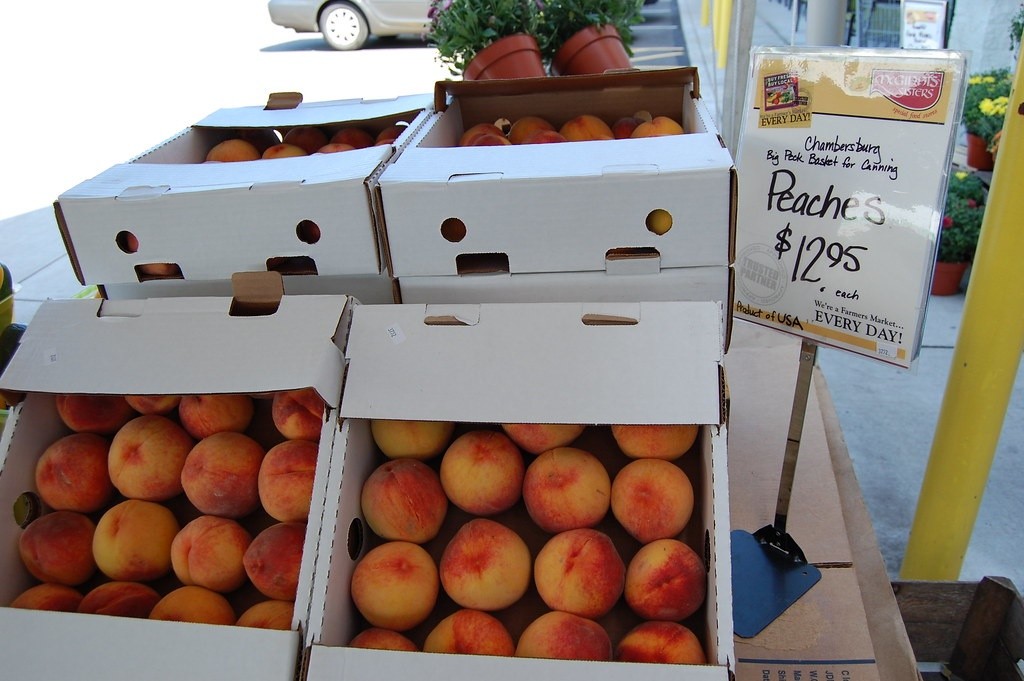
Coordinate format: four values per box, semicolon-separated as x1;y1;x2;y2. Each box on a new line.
7;387;324;630
460;112;687;234
121;121;408;276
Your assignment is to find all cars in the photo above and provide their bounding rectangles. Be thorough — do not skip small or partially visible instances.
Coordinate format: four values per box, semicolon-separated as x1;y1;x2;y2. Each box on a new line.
267;0;452;52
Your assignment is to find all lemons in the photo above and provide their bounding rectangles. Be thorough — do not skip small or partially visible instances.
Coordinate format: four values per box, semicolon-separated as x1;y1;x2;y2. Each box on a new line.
349;419;711;666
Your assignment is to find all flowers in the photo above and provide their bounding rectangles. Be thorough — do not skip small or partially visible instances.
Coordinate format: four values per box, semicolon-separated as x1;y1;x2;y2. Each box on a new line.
936;169;986;263
419;0;646;76
960;69;1012;163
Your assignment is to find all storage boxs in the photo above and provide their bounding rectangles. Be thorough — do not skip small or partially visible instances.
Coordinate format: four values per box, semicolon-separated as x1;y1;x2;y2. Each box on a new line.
0;67;1024;681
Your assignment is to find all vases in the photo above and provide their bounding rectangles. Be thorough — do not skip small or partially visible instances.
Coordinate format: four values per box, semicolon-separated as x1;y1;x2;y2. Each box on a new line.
552;24;631;76
931;262;969;296
463;35;547;81
966;132;995;171
